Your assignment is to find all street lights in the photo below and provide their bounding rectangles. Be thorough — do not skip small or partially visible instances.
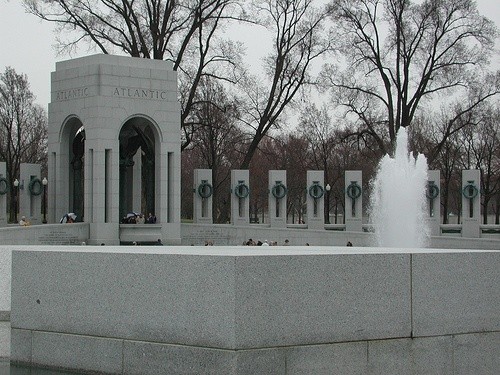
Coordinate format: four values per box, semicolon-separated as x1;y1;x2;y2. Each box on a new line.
42;176;48;224
14;178;19;225
326;183;330;224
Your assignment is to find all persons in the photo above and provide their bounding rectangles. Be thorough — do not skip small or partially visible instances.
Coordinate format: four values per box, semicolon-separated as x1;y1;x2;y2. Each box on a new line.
20;212;79;224
117;212;157;224
79;238;353;247
